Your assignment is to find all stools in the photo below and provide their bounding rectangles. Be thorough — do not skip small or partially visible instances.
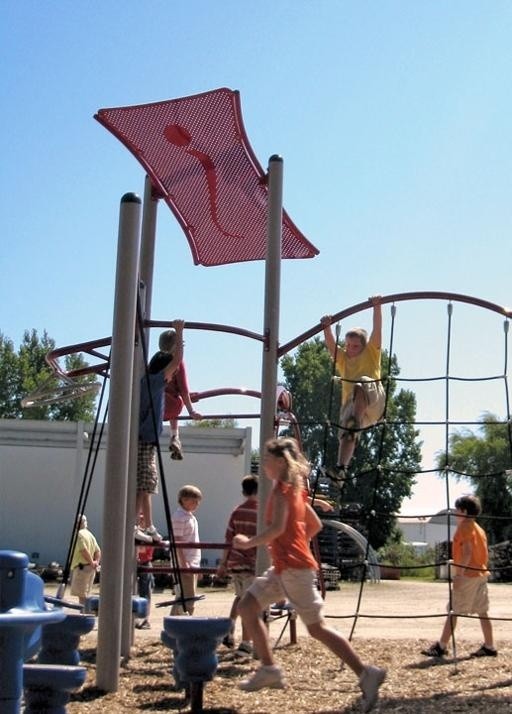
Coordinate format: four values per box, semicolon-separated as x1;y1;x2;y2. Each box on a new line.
23;592;235;712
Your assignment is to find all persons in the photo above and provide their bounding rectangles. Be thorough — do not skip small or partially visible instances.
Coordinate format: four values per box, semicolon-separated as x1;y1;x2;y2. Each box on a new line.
135;535;228;598
170;484;202;614
421;495;498;660
71;513;101;613
218;476;333;656
321;294;388;490
231;434;386;714
133;320;202;543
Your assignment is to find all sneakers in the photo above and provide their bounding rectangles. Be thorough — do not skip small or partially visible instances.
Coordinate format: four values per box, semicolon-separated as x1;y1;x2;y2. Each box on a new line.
144;529;163;542
325;468;347;489
345;416;358;443
357;665;385;711
423;641;446;656
134;526;151;543
470;645;498;657
239;644;251;656
237;667;282;692
170;437;183;461
225;636;234;647
